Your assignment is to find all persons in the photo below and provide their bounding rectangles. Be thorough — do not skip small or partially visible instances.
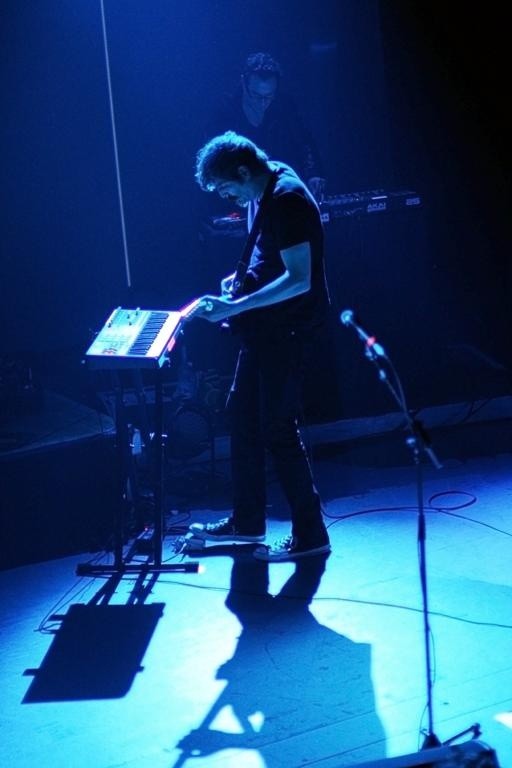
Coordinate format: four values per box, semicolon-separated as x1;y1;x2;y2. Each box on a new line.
187;130;334;563
198;52;329;227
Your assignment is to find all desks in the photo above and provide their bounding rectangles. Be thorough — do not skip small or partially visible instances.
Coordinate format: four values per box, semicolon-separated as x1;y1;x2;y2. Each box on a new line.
0;389;116;465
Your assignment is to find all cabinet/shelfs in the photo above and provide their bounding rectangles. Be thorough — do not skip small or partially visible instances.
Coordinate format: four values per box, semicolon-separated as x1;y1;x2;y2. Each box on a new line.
77;370;198;573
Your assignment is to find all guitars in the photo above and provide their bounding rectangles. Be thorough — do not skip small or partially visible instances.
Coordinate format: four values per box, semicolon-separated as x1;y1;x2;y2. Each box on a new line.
181;277;241;333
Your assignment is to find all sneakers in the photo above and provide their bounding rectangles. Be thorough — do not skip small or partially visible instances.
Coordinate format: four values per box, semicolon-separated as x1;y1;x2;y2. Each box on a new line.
189;518;269;542
252;533;332;561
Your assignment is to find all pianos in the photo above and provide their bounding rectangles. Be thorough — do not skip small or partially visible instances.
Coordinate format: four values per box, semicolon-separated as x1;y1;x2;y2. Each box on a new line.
84;305;185;372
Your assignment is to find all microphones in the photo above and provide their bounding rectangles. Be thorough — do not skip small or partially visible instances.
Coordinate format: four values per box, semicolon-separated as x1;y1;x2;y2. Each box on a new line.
340;309;382;361
